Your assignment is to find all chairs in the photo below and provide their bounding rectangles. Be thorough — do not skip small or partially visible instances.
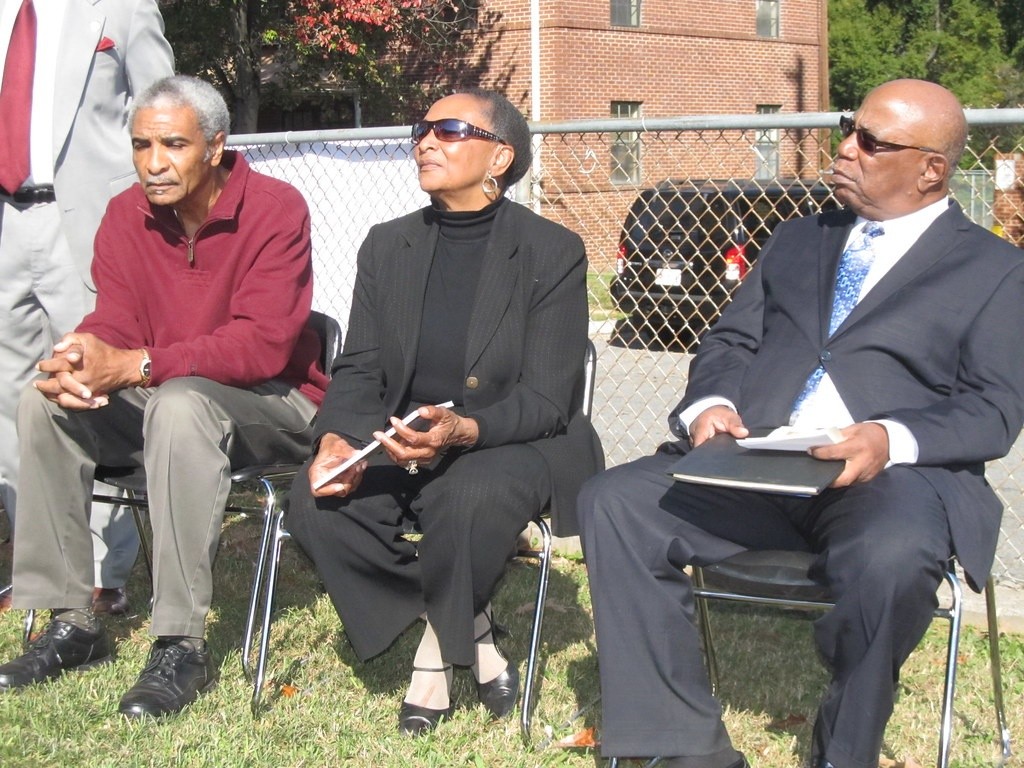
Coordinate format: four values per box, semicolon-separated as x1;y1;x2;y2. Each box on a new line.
605;538;1010;768
21;310;342;683
251;337;596;753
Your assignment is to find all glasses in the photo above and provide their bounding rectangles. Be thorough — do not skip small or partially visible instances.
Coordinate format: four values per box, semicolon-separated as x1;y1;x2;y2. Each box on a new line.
411;117;507;146
839;115;939;157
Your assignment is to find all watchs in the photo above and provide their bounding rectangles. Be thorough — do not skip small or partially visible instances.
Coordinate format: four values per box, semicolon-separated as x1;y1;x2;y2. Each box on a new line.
140;348;151;388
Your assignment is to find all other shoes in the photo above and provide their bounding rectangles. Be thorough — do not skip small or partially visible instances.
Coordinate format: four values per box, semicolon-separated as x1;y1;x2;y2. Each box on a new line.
738;750;751;768
810;742;833;768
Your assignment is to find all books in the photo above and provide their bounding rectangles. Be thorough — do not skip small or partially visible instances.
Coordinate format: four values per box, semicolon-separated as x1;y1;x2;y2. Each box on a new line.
667;426;847;496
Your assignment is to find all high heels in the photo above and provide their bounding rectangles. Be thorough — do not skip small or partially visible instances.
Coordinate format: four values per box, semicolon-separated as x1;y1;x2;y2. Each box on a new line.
399;662;453;738
470;609;519;722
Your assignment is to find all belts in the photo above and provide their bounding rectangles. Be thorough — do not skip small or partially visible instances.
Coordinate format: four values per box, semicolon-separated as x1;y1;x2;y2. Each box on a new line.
0;185;56;203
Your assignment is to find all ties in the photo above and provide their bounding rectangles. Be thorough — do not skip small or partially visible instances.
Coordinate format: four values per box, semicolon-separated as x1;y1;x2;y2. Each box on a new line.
0;0;37;193
788;220;885;430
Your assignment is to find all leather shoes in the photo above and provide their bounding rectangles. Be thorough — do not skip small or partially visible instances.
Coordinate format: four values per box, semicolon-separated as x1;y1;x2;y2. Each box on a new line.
0;620;112;688
118;638;214;719
91;587;128;616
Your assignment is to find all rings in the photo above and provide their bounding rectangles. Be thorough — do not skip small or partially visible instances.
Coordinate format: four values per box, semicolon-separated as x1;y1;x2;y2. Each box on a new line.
404;460;418;475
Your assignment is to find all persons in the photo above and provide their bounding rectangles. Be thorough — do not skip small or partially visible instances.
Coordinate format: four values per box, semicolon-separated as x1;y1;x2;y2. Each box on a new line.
1;73;331;719
0;0;177;644
577;78;1024;768
274;87;605;738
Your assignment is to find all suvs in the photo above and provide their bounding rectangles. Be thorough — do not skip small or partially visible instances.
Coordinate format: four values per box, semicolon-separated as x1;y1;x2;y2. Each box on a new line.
609;178;839;340
